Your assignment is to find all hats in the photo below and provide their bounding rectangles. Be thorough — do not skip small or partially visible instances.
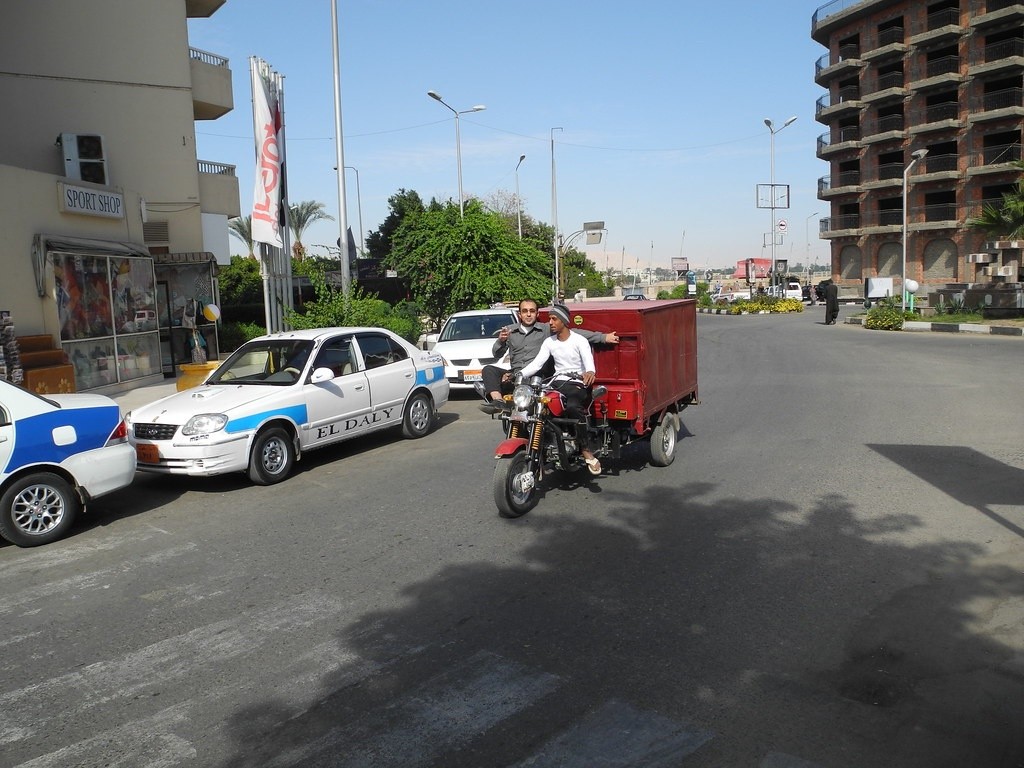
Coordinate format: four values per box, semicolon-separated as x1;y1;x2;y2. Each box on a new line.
548;304;570;326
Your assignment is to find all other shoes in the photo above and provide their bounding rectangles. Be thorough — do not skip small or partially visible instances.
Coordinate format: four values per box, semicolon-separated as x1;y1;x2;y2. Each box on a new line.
479;399;510;414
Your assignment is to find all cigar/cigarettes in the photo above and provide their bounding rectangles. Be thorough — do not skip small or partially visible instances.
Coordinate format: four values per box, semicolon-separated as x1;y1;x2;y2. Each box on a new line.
503;380;506;382
502;326;507;329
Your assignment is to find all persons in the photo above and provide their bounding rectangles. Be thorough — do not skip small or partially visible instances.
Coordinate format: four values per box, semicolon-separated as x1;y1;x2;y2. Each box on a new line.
823;279;839;324
477;297;619;414
502;303;602;475
716;280;723;289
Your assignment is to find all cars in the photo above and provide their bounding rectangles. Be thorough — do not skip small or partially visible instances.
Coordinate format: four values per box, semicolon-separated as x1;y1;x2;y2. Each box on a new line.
0;379;137;548
431;308;521;388
126;327;450;485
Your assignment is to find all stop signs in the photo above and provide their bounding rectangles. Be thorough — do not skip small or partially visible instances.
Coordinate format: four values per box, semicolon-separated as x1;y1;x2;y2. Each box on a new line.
778;220;788;231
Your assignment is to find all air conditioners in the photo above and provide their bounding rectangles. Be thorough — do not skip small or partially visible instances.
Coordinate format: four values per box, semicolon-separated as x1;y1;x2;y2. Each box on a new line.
57;131;109;186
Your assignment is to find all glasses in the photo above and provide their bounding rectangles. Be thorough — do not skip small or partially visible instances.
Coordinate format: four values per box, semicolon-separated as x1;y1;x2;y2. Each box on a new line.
522;308;538;314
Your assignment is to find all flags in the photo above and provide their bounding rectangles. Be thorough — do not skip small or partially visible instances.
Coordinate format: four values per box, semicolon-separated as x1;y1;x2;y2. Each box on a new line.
336;227;357;264
252;64;285;251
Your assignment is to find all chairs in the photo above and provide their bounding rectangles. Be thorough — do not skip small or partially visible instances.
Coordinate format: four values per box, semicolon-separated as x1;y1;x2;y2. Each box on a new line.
460;324;479;337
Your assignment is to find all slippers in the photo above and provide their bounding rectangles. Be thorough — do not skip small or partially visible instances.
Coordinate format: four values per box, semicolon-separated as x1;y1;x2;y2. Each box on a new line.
585;458;601;475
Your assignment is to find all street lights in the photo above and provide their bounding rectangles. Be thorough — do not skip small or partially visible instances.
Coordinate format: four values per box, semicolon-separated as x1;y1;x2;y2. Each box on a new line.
333;166;363;258
762;117;796;298
513;154;527;246
426;89;488;222
902;149;929;319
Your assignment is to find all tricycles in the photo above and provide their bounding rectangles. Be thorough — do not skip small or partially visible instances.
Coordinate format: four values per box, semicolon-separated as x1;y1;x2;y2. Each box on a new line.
472;294;701;517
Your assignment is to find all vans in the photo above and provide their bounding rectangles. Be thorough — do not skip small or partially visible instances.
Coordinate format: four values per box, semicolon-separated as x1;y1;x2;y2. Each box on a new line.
762;283;803;303
